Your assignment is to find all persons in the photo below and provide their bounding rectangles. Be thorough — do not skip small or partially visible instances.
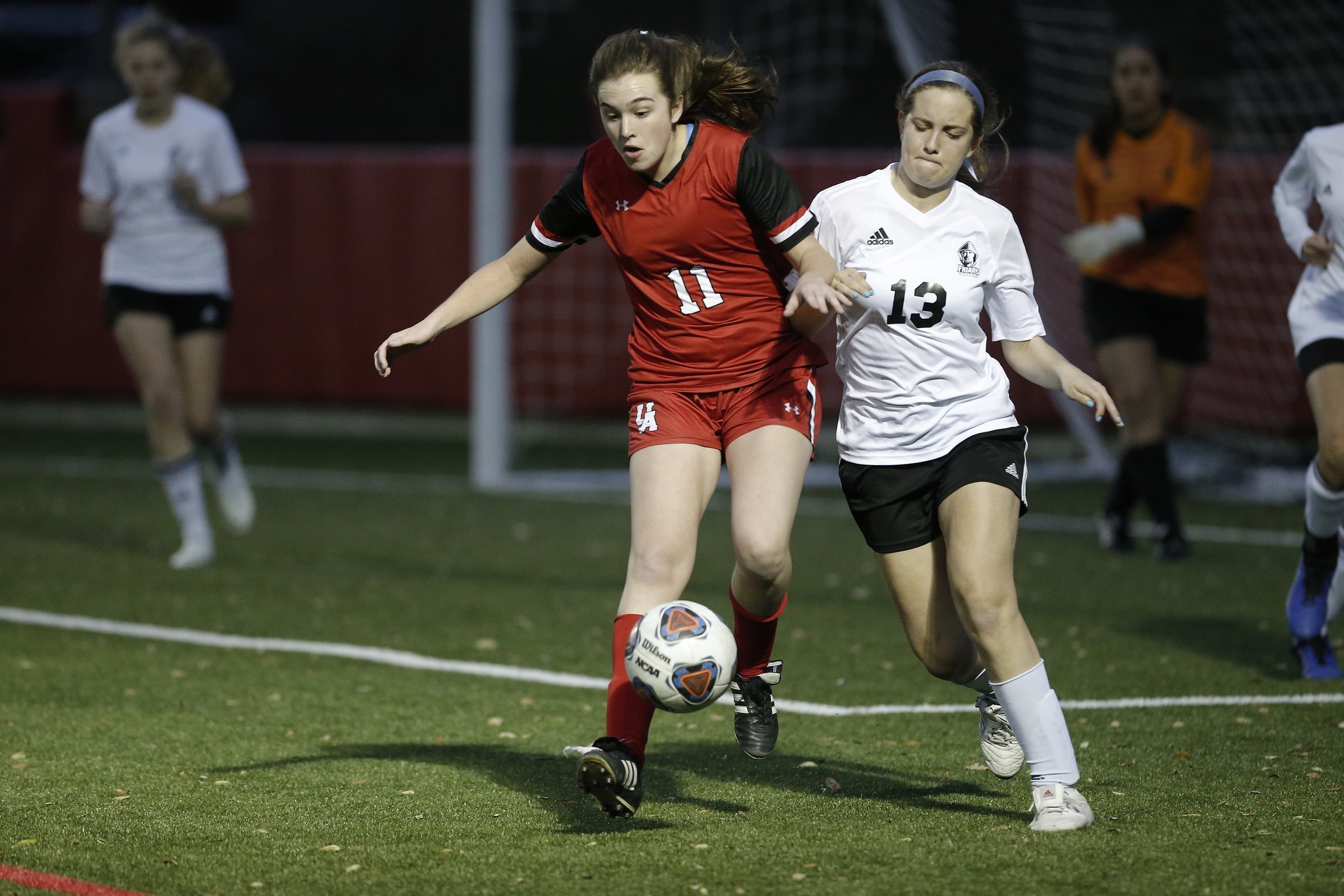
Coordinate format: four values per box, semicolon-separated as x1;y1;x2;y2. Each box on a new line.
1062;39;1211;559
1273;119;1344;690
370;32;875;816
76;4;259;564
785;61;1126;835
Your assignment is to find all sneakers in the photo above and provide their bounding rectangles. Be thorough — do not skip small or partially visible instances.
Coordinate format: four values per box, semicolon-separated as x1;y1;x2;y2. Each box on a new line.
1290;634;1341;677
171;517;220;572
200;439;257;530
1028;784;1096;833
1097;510;1135;552
576;737;644;820
975;693;1025;780
1154;512;1191;558
730;660;784;759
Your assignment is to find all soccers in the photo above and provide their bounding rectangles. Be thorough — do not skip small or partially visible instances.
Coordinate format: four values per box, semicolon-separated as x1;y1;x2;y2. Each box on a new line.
623;600;738;714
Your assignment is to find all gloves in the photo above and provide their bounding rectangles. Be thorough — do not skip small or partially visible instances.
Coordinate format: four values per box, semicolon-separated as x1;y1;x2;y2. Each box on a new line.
1063;218;1141;272
